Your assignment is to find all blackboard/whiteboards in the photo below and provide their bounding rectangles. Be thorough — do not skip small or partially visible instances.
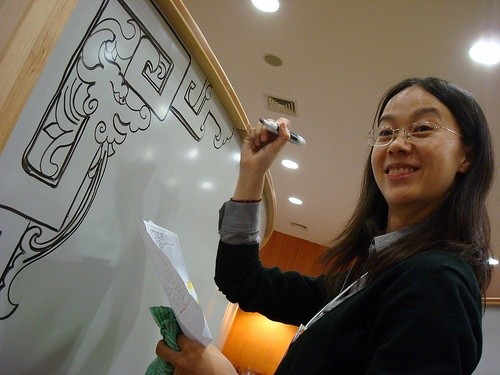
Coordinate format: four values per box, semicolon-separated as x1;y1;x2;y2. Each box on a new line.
0;0;277;375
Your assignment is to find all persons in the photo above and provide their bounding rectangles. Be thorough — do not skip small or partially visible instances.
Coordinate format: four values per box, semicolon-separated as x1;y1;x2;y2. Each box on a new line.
155;76;493;375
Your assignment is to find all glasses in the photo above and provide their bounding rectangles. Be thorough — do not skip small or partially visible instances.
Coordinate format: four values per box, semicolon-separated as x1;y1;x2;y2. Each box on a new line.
365;120;463;146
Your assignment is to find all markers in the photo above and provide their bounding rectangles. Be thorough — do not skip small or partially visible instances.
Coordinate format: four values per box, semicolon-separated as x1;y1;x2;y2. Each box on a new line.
259;118;306;147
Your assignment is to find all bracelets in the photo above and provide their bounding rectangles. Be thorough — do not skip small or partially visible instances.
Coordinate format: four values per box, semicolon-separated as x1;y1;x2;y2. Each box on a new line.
230;197;263;203
